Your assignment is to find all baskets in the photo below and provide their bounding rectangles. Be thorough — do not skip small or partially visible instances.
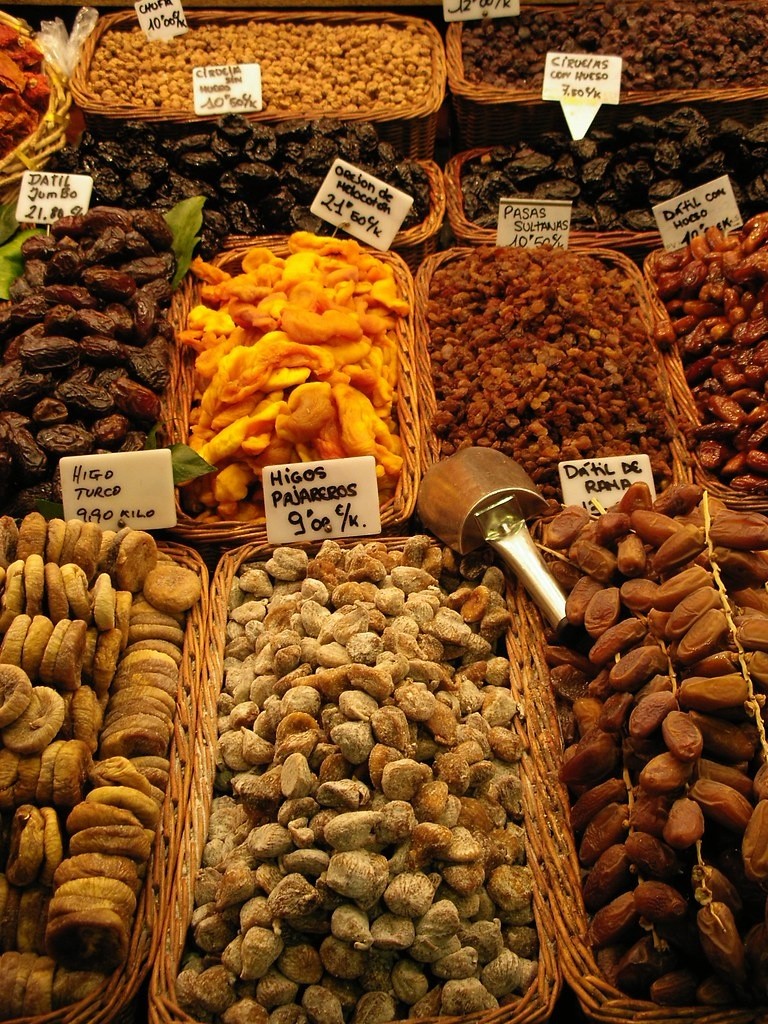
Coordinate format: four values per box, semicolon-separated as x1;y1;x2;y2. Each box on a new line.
0;0;768;1024
